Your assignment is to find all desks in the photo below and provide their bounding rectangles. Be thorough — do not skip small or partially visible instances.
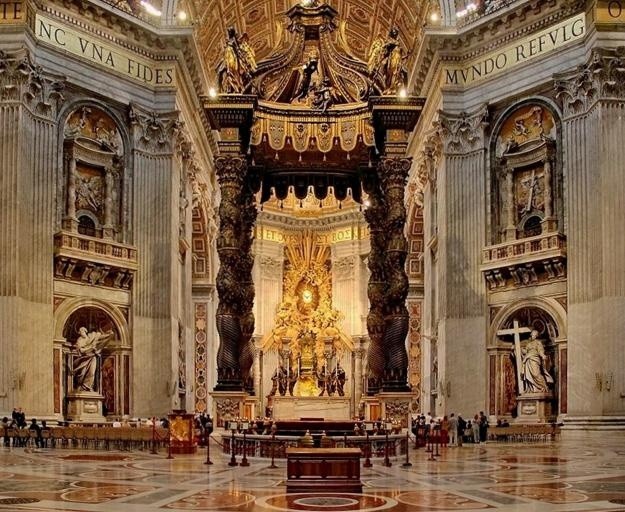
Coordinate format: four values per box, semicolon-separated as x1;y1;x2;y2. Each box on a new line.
285;446;363;493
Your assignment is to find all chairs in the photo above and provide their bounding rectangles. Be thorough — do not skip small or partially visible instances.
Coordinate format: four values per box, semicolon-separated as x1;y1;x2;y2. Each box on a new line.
0;427;153;451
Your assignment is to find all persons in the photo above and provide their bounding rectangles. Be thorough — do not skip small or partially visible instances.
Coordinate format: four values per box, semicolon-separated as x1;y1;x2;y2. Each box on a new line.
112;419;141;427
224;27;409;116
497;105;546;154
72;327;118;394
496;418;509;442
147;416;169;428
67;107;120;149
1;406;49;449
411;411;490;449
375;416;386;452
80;173;99;213
510;328;555;395
193;408;212;446
